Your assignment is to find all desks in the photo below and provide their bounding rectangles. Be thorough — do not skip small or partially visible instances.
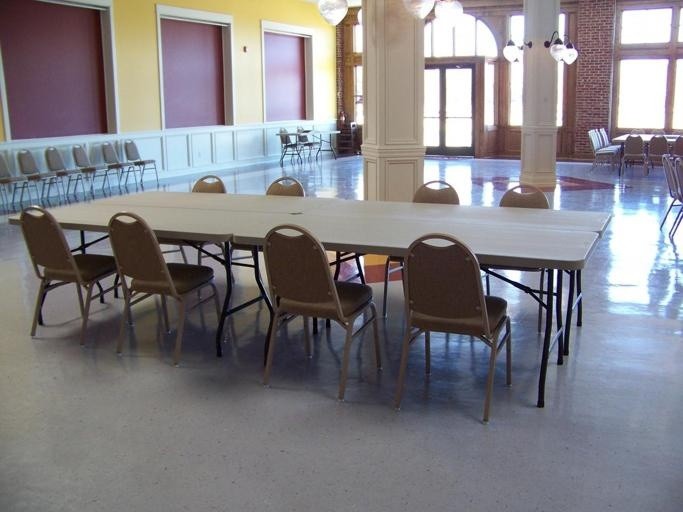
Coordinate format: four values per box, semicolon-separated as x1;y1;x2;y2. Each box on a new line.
6;187;615;411
276;130;342;165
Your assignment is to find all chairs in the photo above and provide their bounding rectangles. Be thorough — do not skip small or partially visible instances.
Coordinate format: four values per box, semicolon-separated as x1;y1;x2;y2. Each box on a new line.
20;173;306;367
472;184;563;334
586;126;683;244
261;223;383;408
0;130;163;215
278;125;320;166
382;180;460;321
391;232;512;422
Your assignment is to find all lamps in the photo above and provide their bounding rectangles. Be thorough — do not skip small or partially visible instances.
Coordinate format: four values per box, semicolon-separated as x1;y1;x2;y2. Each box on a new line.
561;33;578;65
501;38;532;62
407;1;438;20
317;0;350;28
434;1;463;27
543;29;567;63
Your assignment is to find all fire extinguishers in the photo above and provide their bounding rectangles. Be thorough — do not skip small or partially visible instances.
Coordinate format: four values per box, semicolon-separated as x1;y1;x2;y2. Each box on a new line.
339;111;347;128
